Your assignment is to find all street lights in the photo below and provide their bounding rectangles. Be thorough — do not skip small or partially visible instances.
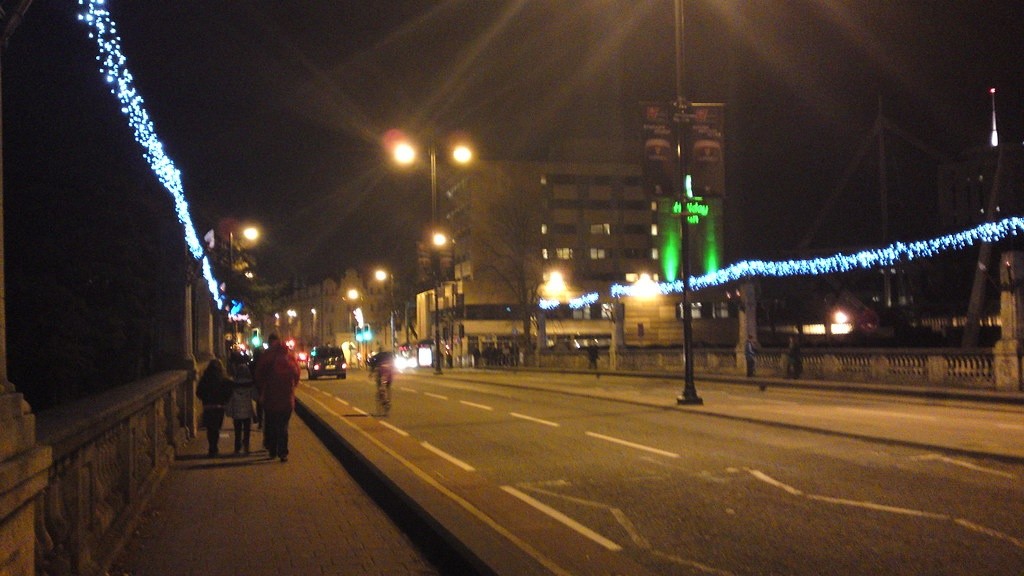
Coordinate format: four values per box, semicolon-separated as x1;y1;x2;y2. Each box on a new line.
230;227;259;354
434;235;456;368
396;129;472;374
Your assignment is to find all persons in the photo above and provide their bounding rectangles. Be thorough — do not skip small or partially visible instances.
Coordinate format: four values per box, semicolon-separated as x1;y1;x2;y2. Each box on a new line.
195;359;254;456
368;344;394;416
224;363;260;456
440;344;518;368
248;332;301;463
744;334;758;377
587;339;600;369
226;344;266;430
786;334;801;379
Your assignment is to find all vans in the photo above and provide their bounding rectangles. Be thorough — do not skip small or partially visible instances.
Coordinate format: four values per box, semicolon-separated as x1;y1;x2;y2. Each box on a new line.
305;345;346;380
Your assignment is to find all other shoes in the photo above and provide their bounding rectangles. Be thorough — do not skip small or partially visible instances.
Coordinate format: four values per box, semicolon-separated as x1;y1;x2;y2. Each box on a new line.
234;447;250;455
268;451;288;462
208;448;219;456
